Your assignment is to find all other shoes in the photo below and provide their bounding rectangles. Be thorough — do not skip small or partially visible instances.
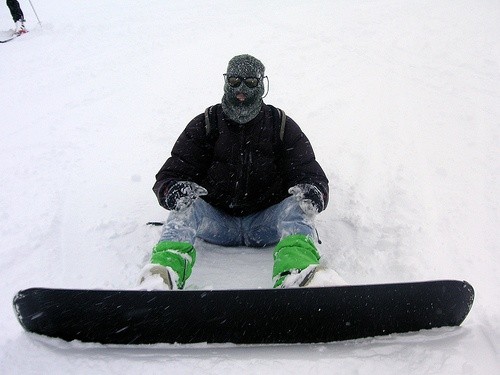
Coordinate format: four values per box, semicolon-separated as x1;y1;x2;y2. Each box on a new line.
14;19;27;36
274;264;317;288
139;264;174;291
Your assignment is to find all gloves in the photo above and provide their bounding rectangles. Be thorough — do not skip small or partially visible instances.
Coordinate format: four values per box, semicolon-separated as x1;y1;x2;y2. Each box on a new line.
287;183;325;215
164;181;207;212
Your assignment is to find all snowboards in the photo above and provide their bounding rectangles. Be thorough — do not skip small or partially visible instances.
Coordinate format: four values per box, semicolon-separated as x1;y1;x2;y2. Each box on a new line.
13;280;475;345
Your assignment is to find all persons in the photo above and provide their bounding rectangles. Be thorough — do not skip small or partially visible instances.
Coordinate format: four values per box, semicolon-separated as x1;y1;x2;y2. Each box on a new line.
138;55;330;290
6;0;27;37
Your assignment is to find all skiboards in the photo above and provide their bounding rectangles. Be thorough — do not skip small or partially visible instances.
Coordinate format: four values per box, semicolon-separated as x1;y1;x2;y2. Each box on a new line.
0;28;29;43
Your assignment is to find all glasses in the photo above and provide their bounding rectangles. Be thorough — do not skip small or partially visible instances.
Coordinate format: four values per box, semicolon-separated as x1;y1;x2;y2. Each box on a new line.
227;73;262;88
